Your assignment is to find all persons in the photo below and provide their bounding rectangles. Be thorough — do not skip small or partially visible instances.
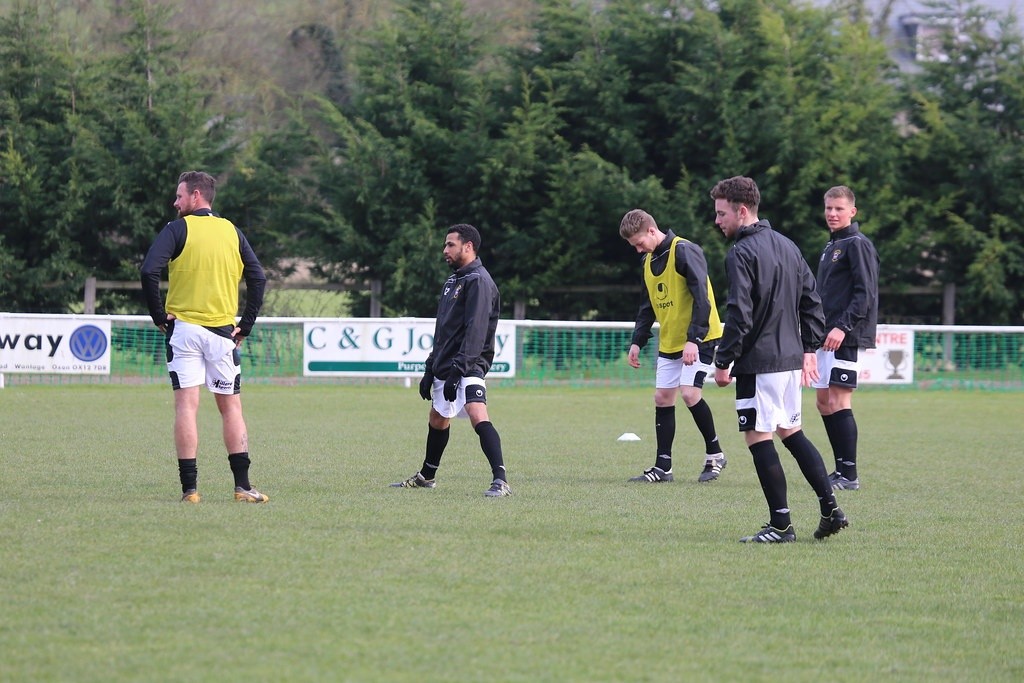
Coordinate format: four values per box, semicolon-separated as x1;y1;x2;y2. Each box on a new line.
621;209;727;484
816;186;879;490
390;225;512;496
711;176;849;542
142;172;269;502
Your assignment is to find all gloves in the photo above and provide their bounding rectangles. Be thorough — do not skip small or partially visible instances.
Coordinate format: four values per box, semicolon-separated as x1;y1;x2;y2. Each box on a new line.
420;370;434;400
444;367;462;403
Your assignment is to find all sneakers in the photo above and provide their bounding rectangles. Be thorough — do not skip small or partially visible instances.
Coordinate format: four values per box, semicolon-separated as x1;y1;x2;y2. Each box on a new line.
390;472;435;488
738;522;797;543
234;485;269;503
182;489;200;503
629;466;673;482
828;471;839;481
485;478;511;497
830;473;860;491
813;507;848;538
698;452;727;482
